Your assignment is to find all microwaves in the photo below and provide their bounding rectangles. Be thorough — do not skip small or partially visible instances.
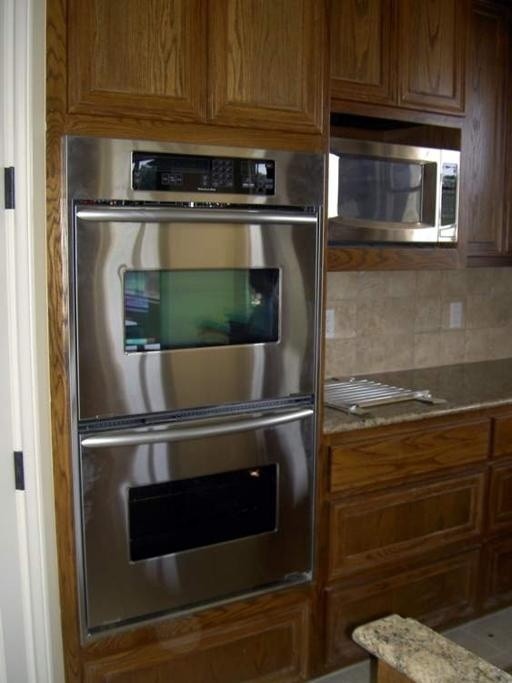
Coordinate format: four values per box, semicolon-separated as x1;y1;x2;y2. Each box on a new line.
324;137;460;241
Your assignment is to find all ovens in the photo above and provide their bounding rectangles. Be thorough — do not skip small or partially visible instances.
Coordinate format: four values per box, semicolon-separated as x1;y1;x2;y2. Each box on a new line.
67;207;316;637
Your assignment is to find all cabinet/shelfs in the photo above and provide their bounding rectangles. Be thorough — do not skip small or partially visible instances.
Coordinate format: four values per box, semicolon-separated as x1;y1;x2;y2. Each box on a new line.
481;400;512;616
309;410;492;675
327;1;465;136
44;0;327;149
383;1;510;272
